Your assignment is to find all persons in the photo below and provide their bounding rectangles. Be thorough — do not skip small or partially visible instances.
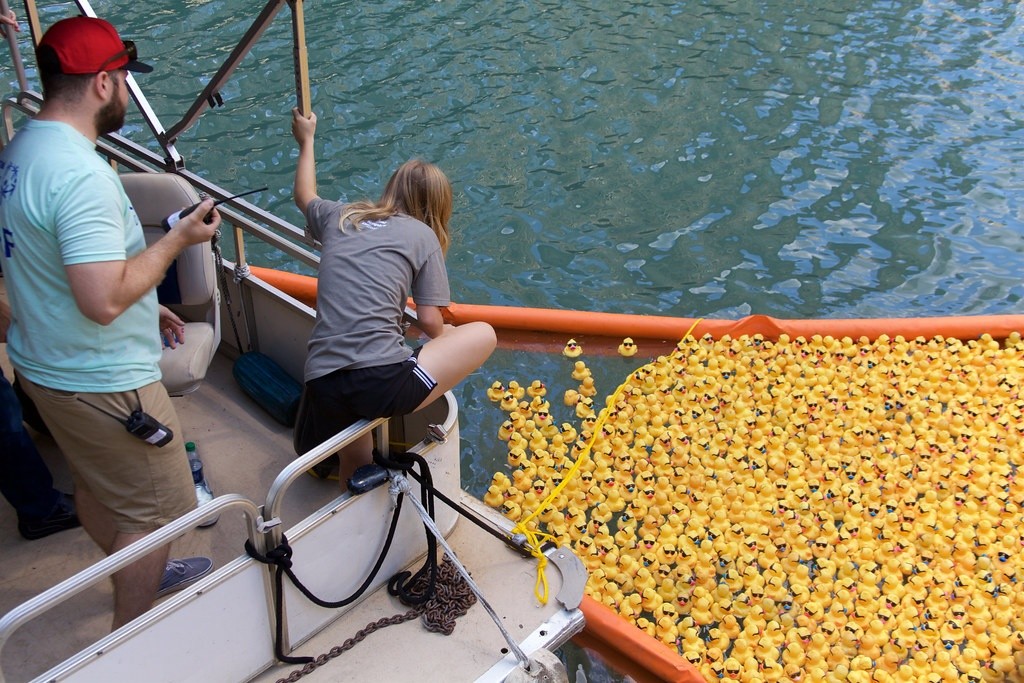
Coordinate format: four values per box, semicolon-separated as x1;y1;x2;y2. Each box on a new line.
0;13;222;635
291;106;497;495
0;10;20;39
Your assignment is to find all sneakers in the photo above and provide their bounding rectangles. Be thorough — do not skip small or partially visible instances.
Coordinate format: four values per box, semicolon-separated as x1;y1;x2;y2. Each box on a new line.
154;556;214;600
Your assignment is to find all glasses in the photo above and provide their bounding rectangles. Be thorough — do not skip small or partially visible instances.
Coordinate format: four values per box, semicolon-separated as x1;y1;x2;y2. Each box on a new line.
96;40;137;75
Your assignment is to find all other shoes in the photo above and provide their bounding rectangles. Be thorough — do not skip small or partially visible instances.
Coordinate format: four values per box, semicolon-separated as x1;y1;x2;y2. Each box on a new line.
18;493;83;539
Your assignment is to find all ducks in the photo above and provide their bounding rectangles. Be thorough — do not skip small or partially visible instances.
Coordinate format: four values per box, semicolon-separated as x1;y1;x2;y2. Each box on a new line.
483;333;1024;683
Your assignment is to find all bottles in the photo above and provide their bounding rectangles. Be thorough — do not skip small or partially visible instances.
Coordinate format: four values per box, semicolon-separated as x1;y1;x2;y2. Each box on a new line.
185;442;199;462
189;459;219;526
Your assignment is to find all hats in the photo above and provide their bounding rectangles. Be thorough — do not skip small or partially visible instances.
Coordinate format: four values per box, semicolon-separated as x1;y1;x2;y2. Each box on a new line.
36;14;154;75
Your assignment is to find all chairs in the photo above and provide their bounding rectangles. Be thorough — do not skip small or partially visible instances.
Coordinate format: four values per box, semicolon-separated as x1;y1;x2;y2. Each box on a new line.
118;173;221;398
